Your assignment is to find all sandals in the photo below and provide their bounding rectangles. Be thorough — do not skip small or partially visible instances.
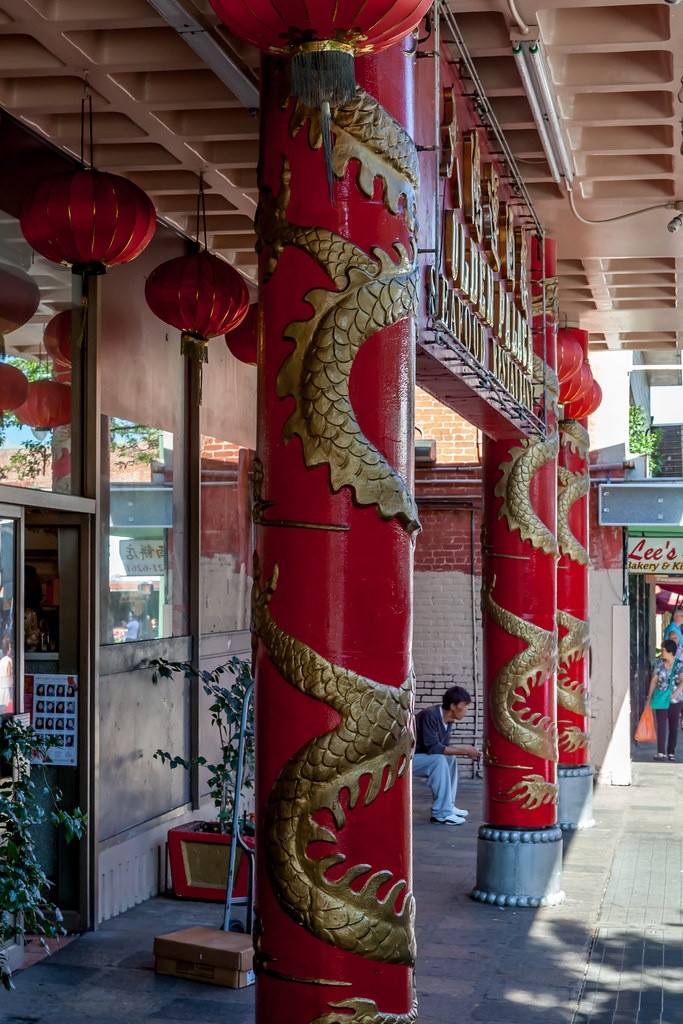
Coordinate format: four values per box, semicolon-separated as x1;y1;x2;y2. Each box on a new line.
653;751;667;759
669;753;676;761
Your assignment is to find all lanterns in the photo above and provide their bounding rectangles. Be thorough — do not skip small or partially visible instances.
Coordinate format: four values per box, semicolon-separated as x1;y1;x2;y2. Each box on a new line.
42;307;73;371
559;379;602;424
0;261;40;360
17;165;158;348
143;248;250;406
558;363;595;407
224;301;259;367
212;0;436;201
0;362;28;426
532;327;585;386
13;378;72;476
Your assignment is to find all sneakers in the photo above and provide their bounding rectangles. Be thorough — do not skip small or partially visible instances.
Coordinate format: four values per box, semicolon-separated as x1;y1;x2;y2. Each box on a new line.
430;813;467;826
451;804;469;817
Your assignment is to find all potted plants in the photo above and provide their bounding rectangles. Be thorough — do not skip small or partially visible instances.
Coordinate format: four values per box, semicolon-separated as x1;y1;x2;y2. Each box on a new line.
128;653;257;901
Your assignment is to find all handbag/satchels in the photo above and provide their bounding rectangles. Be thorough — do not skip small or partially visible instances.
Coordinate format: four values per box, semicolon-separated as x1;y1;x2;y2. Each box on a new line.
649;687;672;709
634;705;657;743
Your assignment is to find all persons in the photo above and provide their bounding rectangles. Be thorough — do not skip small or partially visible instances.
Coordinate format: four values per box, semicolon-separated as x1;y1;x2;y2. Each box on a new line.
413;684;482;827
55;685;65;698
35;701;74;714
108;616;153;642
46;684;55;697
36;718;74;731
36;684;45;697
0;637;13;713
66;686;75;697
35;733;73;747
644;608;683;762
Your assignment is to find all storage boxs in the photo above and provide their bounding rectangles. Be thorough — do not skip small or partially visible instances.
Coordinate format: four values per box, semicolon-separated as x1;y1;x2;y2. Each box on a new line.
152;926;255;989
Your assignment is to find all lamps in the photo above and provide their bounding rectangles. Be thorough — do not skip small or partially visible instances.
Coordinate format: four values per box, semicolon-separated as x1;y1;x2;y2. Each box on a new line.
510;26;578;183
